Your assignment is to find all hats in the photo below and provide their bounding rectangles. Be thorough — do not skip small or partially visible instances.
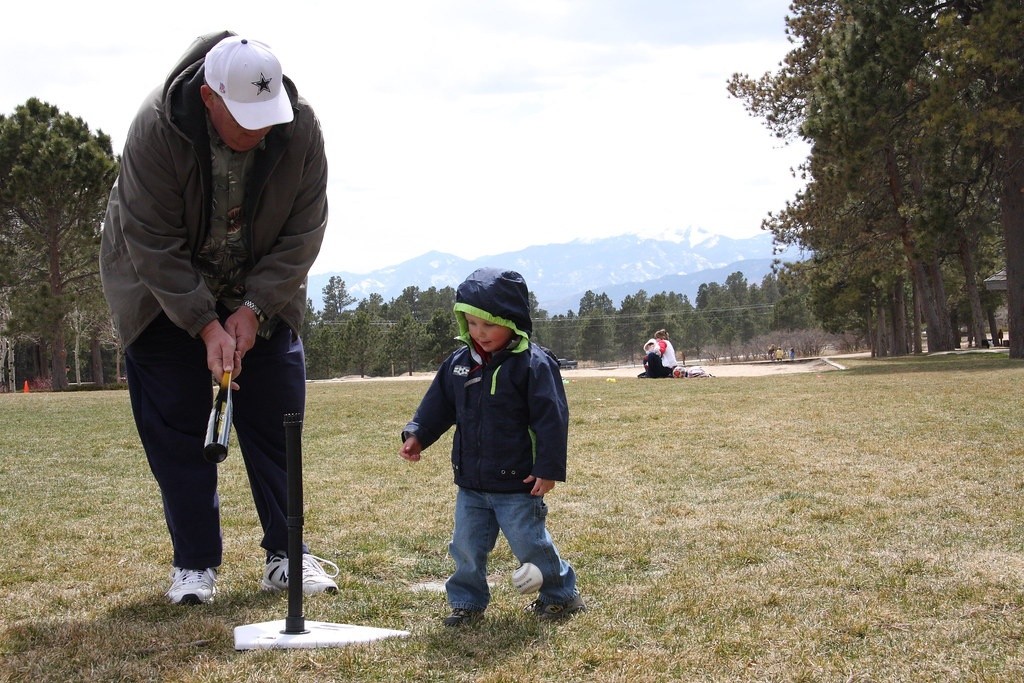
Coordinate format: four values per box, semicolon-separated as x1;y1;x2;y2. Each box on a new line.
204;35;294;130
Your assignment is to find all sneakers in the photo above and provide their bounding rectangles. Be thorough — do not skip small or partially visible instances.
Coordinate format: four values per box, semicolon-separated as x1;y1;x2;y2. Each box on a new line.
262;541;339;596
164;565;218;605
524;593;587;620
443;609;485;626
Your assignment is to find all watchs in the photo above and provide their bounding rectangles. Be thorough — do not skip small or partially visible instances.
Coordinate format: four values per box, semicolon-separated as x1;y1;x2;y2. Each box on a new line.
242;300;265;323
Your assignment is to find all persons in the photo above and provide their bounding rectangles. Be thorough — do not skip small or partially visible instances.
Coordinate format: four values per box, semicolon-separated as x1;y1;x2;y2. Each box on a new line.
789;347;795;365
99;29;340;604
981;337;994;349
399;266;585;630
768;344;782;366
997;329;1003;346
642;329;710;379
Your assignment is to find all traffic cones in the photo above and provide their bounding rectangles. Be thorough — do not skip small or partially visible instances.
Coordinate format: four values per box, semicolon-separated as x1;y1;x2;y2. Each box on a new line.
23;380;29;393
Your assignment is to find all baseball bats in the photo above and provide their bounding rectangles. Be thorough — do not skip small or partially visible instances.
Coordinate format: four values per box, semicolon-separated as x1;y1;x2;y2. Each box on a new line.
203;361;233;463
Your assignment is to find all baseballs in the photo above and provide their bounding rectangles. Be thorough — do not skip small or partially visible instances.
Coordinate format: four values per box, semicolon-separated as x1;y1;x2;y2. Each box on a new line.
510;563;545;595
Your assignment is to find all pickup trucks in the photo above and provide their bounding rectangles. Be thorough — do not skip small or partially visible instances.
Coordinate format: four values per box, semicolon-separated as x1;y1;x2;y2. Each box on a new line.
557;358;578;370
